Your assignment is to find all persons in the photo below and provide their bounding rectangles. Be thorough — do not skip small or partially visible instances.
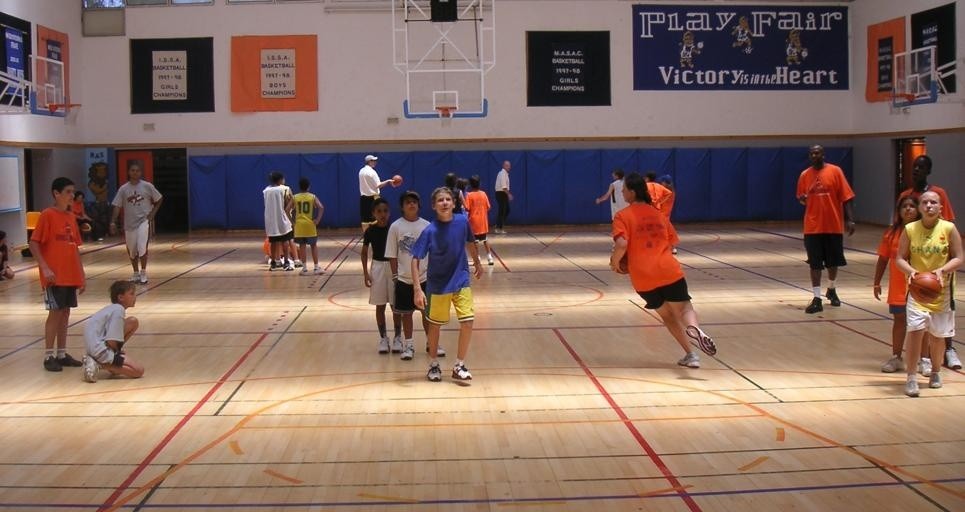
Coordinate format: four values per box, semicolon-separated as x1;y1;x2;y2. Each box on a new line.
608;172;719;368
895;190;960;392
0;230;15;282
732;16;754;54
384;189;446;360
642;172;672;211
495;160;515;234
70;190;92;242
596;168;625;252
786;29;808;66
262;173;286;267
455;178;468;193
677;31;703;68
361;197;403;354
28;177;84;372
465;174;494;266
656;174;678;255
358;154;396;232
262;172;294;271
873;195;935;378
284;178;326;276
280;176;303;267
82;179;99;241
444;172;466;213
90;178;110;241
109;160;163;285
82;280;145;383
797;145;856;315
892;154;963;370
410;186;485;386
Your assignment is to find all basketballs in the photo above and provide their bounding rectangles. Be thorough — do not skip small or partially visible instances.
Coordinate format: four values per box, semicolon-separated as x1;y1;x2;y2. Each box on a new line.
610;250;631;275
81;222;92;232
909;272;942;303
393;174;402;184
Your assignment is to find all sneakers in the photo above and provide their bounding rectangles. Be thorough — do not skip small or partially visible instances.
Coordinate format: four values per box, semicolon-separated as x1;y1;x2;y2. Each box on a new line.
929;371;941;386
678;353;701;368
424;343;446;356
687;325;717;356
313;267;326;275
298;269;309;276
128;276;138;283
426;361;441;381
805;297;823;313
488;257;495;265
269;261;276;271
83;354;98;383
452;361;472;380
55;353;83;367
905;375;919;396
881;355;905;371
294;260;303;266
140;274;149;285
391;336;402;353
919;356;932;376
379;337;390;354
944;347;962;368
43;355;63;371
400;341;414;360
825;288;841;306
283;260;290;271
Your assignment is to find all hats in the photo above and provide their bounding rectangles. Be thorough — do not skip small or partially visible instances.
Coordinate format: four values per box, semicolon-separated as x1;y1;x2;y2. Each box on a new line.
365;155;379;162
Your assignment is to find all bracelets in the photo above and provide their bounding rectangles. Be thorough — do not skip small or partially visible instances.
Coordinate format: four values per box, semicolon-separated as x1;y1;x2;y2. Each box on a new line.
873;285;880;288
848;221;855;224
110;223;115;226
